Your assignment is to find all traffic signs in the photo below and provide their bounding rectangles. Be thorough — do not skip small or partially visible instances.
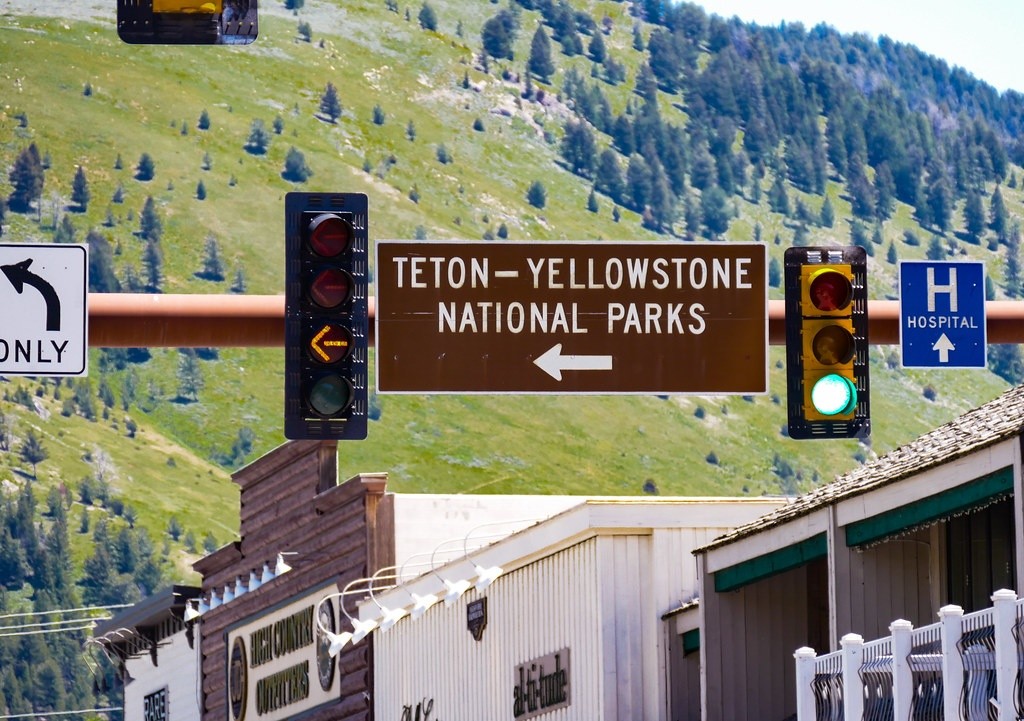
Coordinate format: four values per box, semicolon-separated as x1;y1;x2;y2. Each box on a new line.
376;240;771;395
899;261;987;370
0;243;90;377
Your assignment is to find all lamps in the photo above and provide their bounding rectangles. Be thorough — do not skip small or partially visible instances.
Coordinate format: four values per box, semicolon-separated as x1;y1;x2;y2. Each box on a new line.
273;550;332;579
209;586;233;611
399;547;476;622
316;585;397;657
234;573;262;598
248;565;298;593
183;597;209;622
222;580;247;605
431;532;511;607
369;559;453;632
341;573;422;645
81;626;172;699
260;559;313;585
197;592;223;617
465;517;543;594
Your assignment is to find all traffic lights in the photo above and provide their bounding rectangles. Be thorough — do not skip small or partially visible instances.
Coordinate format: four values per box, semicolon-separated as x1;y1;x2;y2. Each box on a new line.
783;246;871;440
286;191;369;439
